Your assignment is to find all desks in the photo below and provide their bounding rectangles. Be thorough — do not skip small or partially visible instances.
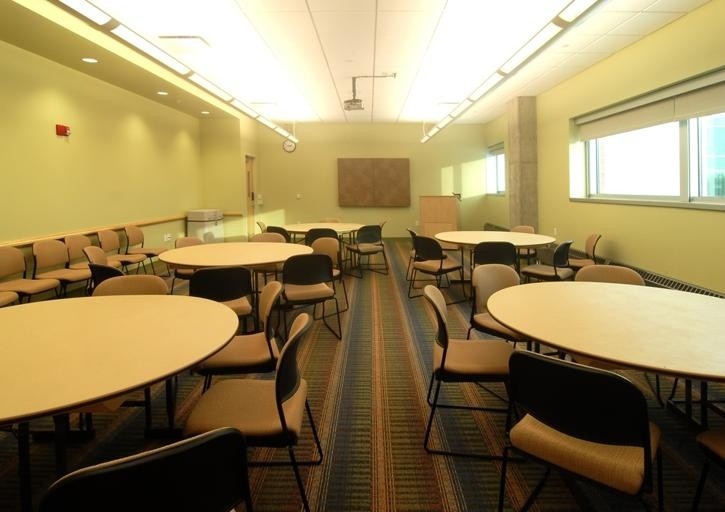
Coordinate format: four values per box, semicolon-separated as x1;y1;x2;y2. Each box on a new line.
2;291;242;506
419;195;461;250
481;277;724;510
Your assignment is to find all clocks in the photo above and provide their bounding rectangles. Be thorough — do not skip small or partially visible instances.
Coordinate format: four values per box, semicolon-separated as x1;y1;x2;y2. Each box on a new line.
282;139;296;153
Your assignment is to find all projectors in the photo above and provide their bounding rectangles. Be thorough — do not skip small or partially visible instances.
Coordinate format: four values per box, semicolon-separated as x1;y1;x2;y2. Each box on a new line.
344;99;364;110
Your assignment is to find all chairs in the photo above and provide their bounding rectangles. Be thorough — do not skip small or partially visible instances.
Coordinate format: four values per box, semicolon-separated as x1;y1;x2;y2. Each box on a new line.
407;227;617;308
497;347;668;509
180;311;328;510
192;280;288;394
573;263;648;288
419;283;530;465
41;424;257;511
465;262;526;355
2;220;389;345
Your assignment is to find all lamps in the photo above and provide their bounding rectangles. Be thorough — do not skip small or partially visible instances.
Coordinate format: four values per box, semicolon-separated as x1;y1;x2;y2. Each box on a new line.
57;0;299;145
420;1;599;144
